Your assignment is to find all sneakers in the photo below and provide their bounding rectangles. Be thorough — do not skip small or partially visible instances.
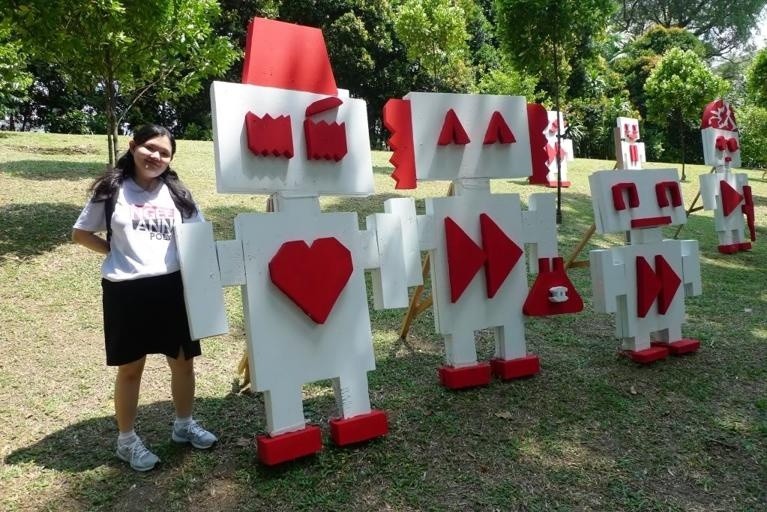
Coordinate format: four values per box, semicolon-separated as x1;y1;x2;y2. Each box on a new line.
172;421;218;449
116;437;161;472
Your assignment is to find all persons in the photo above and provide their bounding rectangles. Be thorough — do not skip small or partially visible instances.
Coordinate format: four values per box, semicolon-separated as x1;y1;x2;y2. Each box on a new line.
70;122;219;472
